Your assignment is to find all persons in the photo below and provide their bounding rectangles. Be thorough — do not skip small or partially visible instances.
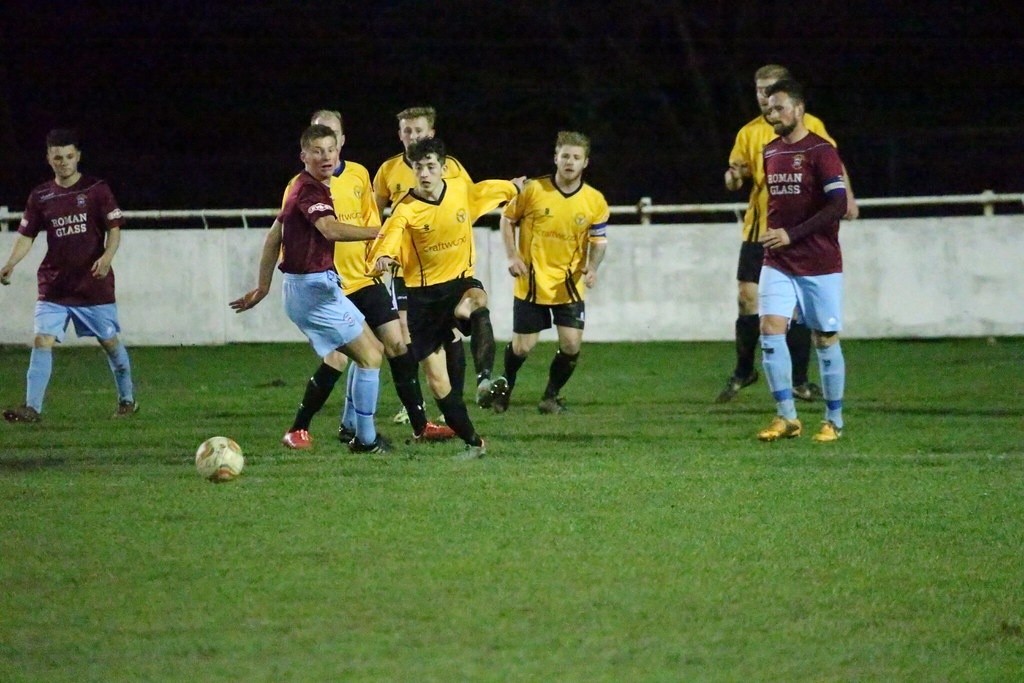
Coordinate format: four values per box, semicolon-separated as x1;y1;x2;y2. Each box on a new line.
228;125;395;455
716;64;858;402
494;133;610;414
365;139;527;459
757;82;848;440
0;129;141;422
283;110;460;448
373;108;473;424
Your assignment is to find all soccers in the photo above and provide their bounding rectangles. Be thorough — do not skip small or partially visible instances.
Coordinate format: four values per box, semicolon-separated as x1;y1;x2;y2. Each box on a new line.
194;436;244;483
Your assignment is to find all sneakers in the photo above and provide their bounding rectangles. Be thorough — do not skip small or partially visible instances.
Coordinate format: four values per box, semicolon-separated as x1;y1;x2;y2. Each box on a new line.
336;422;393;445
792;383;822;402
756;415;802;441
1;406;43;426
535;395;573;418
410;422;456;441
436;412;450;425
447;438;487;466
282;428;314;452
348;433;398;457
111;397;141;420
715;369;759;404
393;400;428;426
812;418;842;442
494;374;519;415
474;376;506;410
